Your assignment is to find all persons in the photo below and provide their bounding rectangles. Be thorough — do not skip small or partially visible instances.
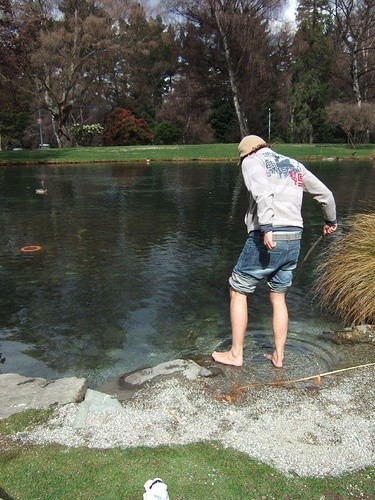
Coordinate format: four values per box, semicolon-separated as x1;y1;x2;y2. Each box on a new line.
211;135;338;367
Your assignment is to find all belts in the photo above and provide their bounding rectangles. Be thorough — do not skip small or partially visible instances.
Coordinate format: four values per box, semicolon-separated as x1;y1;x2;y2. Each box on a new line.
246;229;303;242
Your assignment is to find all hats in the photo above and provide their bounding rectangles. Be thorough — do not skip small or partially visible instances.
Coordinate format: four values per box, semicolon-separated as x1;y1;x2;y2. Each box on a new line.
236;135;269;167
